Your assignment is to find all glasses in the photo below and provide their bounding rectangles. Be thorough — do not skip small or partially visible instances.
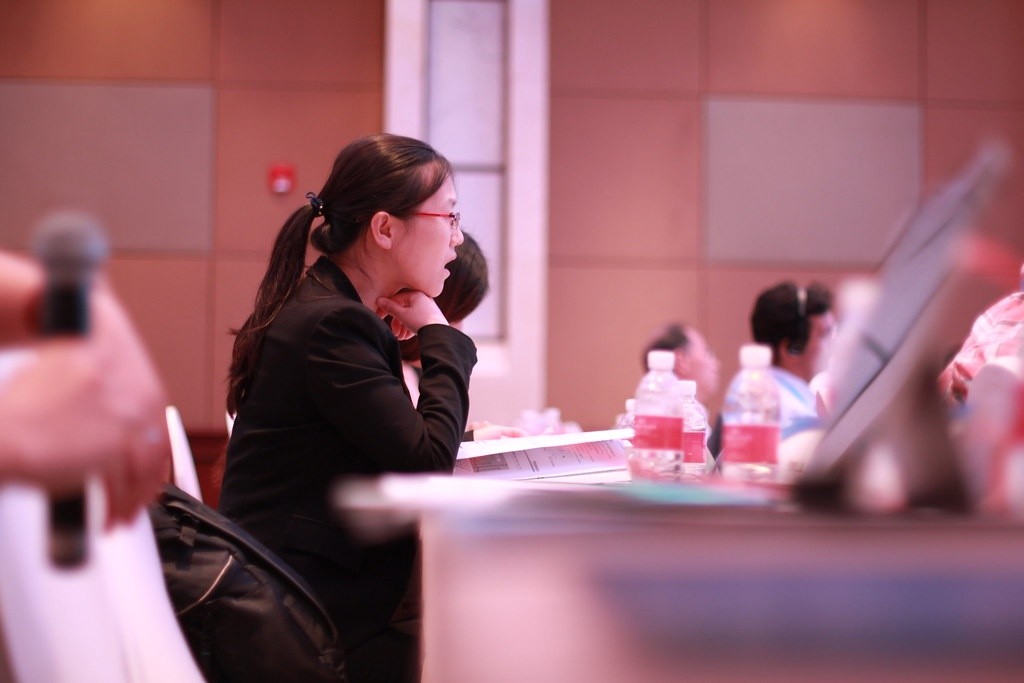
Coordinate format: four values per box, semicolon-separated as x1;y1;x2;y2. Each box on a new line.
356;204;460;234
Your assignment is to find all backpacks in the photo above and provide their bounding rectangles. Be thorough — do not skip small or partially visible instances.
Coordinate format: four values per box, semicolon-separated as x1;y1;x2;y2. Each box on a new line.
147;483;350;683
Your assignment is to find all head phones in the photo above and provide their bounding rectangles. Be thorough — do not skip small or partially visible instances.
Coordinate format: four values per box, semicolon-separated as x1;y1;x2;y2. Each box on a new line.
786;287;812;357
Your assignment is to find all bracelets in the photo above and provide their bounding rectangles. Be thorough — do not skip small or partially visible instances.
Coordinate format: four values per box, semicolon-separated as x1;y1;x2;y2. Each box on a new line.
956;233;1024;292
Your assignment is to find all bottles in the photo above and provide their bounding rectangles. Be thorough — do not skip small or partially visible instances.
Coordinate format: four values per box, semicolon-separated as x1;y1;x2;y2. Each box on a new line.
720;343;783;485
613;350;708;482
514;405;583;436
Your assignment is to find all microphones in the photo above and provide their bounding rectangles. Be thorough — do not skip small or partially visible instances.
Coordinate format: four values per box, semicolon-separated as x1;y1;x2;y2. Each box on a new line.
29;212;111;564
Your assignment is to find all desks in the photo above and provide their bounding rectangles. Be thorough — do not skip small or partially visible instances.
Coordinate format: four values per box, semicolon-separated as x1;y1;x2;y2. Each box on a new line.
328;472;1023;682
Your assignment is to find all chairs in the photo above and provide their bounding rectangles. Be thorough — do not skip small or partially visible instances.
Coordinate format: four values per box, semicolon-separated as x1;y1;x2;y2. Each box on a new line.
150;485;348;682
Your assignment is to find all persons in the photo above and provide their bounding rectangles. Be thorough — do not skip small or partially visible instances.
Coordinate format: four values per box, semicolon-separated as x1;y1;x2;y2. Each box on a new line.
822;145;1024;524
0;246;172;534
721;276;837;438
217;131;529;683
382;227;487;441
642;323;722;403
